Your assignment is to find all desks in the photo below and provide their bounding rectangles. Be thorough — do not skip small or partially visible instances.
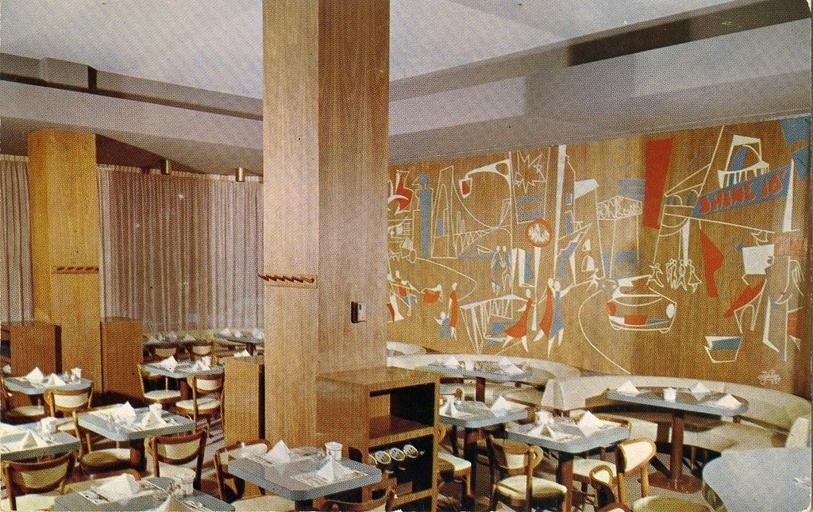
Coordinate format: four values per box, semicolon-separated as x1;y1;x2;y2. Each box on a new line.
606;387;749;493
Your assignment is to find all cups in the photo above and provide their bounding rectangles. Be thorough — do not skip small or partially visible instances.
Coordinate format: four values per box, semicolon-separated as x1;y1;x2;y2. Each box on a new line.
71;367;82;379
369;444;418;470
40;416;56;433
174;467;197;496
202;356;211;366
663;386;676;400
149;402;162;418
325;441;343;461
535;408;550;427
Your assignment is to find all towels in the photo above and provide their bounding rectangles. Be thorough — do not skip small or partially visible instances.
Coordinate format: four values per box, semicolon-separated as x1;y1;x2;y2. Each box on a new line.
712;392;742;410
692;383;709;400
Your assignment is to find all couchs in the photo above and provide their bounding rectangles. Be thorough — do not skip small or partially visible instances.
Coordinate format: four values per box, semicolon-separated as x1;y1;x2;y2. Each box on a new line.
543;375;812;512
